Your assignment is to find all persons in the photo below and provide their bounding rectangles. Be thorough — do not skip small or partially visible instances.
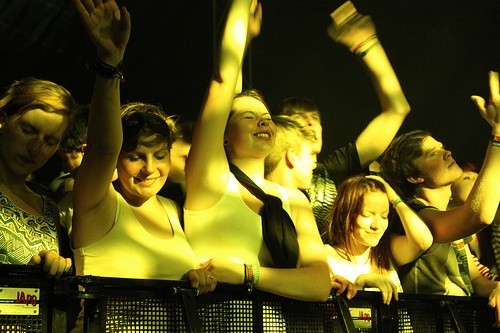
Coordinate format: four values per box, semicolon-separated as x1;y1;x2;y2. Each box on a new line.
0;0;500;333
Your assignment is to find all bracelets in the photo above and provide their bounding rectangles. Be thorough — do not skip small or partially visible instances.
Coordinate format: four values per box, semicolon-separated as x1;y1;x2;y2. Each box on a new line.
247;263;254;292
355;40;380;59
489;140;500;147
95;54;124;79
244;264;248;283
392;199;402;208
350;33;378;53
252;263;259;289
489;134;500;142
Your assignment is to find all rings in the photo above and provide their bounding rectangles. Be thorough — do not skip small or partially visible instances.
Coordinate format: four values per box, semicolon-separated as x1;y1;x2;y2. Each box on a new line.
204;275;214;279
